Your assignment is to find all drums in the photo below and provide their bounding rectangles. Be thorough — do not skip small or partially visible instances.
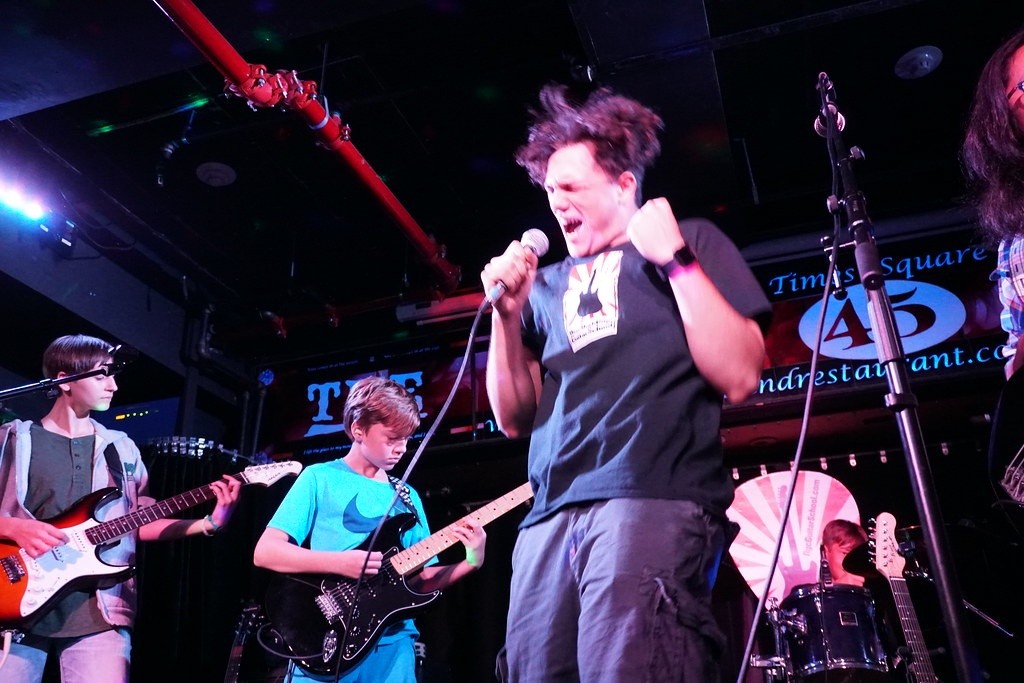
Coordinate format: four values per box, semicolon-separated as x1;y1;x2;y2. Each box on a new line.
773;584;890;683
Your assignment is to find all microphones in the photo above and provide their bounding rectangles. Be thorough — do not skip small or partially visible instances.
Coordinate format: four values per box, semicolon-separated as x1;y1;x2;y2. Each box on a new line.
813;72;845;137
485;228;549;305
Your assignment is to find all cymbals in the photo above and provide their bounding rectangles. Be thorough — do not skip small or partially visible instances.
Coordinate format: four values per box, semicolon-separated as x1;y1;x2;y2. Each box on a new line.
896;525;923;531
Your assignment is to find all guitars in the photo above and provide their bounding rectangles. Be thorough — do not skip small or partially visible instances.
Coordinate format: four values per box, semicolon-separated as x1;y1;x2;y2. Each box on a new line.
868;511;941;683
223;600;264;683
265;482;534;683
0;460;303;647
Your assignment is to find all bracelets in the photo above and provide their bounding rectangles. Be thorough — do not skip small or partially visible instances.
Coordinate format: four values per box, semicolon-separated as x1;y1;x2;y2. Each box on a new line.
202;515;222;537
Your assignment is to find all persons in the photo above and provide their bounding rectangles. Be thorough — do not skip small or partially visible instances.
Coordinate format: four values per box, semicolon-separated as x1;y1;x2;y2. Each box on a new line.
963;34;1024;385
481;84;770;683
254;375;486;683
0;335;242;683
784;520;911;683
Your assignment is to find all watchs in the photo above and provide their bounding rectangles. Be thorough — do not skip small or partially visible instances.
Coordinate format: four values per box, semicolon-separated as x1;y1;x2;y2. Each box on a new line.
661;244;696;277
